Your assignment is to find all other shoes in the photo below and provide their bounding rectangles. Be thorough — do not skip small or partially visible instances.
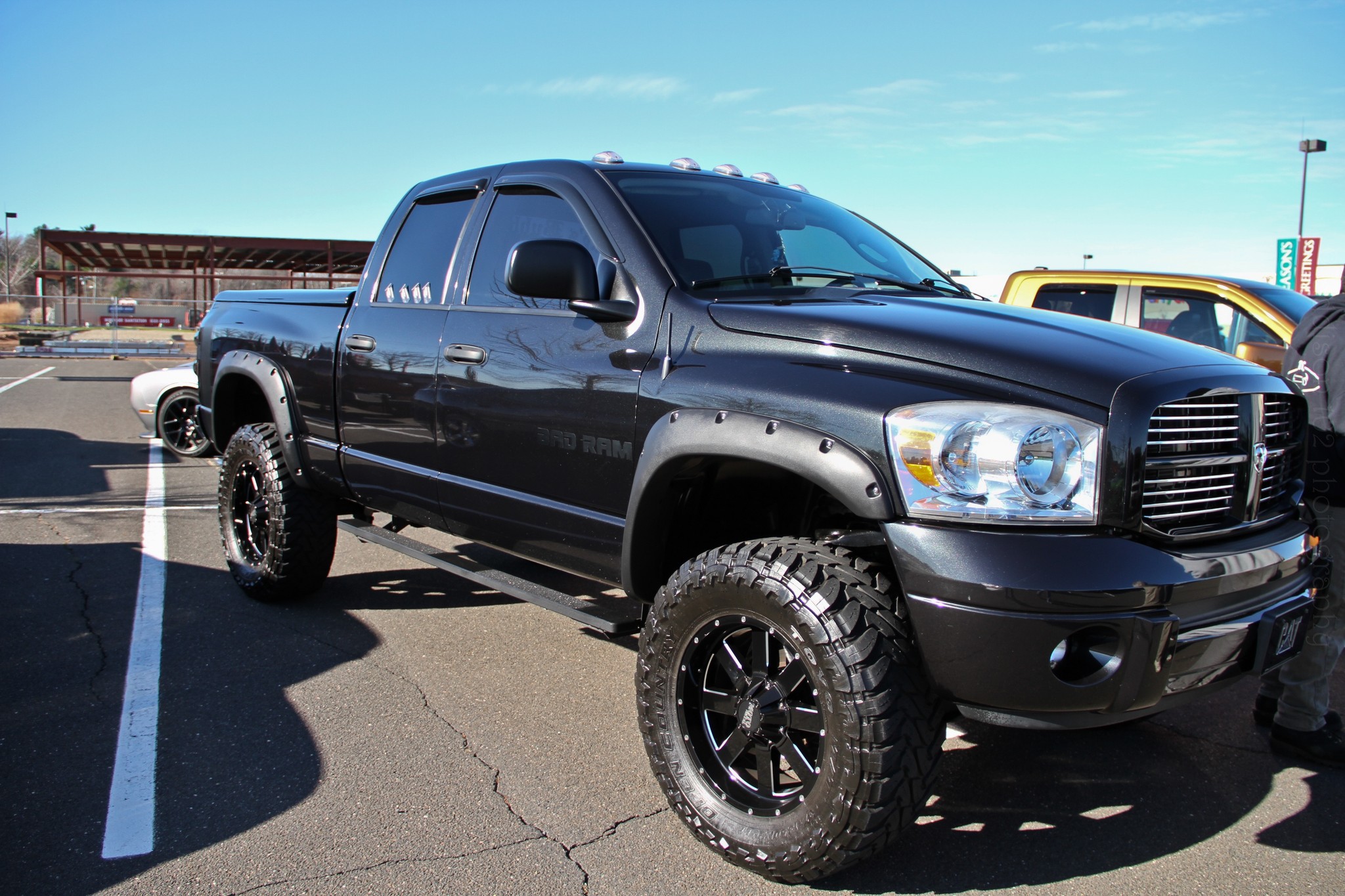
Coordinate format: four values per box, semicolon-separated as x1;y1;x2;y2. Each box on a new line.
1268;724;1345;771
1254;694;1342;732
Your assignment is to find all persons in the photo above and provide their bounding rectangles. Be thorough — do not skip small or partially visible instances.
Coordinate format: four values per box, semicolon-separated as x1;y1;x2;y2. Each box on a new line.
1252;262;1345;772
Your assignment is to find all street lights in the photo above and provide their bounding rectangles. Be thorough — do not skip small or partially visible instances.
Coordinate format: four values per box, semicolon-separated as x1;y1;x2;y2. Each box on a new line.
1297;139;1328;236
5;212;18;302
1083;254;1093;270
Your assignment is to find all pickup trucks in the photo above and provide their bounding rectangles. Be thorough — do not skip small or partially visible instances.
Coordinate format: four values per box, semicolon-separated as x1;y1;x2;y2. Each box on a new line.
189;149;1338;890
996;264;1318;378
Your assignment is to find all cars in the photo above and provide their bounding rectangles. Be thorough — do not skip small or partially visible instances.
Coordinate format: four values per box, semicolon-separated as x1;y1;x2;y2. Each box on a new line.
129;359;211;458
